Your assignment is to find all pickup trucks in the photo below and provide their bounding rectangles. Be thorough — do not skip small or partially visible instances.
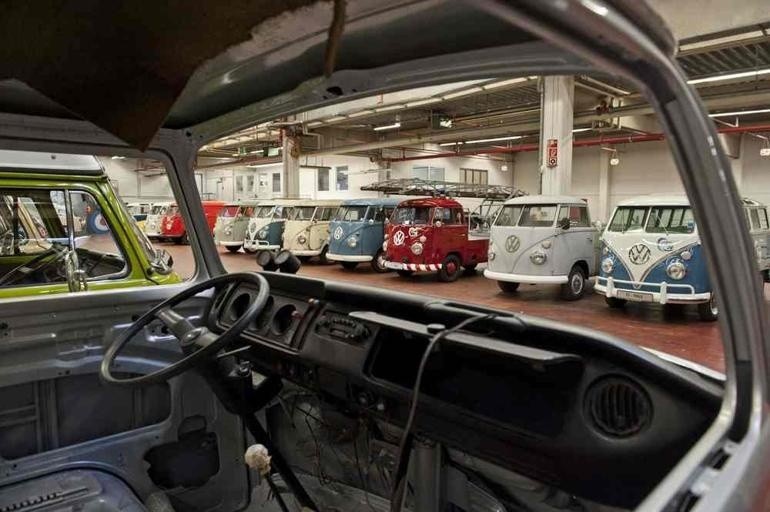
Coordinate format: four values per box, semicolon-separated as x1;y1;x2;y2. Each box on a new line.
477;193;602;299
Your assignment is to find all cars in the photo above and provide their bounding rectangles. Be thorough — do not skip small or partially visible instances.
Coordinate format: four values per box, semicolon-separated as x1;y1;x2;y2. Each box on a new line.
594;194;770;321
124;199;398;272
0;153;180;296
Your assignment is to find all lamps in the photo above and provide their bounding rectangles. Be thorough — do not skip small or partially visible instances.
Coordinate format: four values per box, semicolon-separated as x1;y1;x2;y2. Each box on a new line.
610;151;620;166
760;139;770;156
500;164;508;172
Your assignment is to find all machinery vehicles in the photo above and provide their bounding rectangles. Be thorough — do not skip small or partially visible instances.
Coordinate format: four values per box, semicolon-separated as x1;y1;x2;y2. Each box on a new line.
361;175;529;284
